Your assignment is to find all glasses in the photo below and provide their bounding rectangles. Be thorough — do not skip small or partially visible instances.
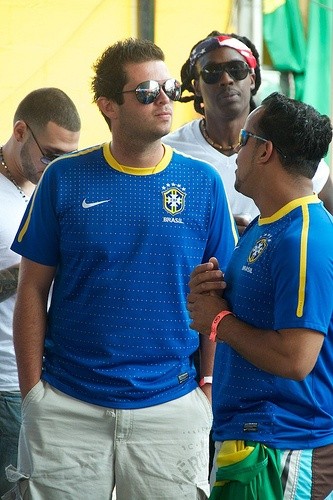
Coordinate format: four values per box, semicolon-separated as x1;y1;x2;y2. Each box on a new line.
104;78;182;106
197;59;252;85
26;123;60;166
238;129;289;161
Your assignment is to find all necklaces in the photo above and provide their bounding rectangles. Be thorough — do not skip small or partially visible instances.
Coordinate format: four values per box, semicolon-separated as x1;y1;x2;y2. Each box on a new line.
202;117;239;150
0;146;28;203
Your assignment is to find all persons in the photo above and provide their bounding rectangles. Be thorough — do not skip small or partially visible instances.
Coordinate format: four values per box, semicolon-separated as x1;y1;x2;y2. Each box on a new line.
12;38;240;500
186;91;333;500
0;87;80;500
161;30;332;239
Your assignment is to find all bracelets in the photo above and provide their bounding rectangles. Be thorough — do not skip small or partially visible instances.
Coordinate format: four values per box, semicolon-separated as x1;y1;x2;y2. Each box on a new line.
208;310;237;344
199;376;213;388
